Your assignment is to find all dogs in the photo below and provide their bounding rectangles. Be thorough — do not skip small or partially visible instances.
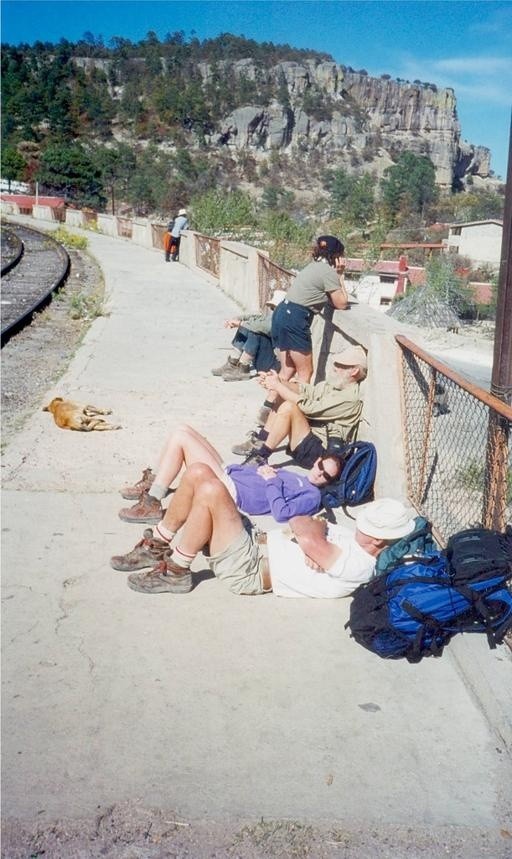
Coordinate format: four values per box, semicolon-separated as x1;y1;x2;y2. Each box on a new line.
42;398;123;431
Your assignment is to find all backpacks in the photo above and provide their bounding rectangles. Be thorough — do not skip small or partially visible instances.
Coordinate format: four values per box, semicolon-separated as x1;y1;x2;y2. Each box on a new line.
375;516;434;574
349;528;510;664
321;441;376;507
168;217;175;232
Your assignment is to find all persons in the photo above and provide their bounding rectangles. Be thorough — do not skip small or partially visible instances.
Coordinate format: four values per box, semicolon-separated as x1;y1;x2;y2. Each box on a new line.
164;208;189;262
118;424;343;523
258;235;350;423
241;344;368;470
110;462;415;602
210;289;288;382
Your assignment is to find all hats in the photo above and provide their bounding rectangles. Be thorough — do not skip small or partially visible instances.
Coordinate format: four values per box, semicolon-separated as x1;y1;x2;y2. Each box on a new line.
317;236;345;252
331;345;367;369
354;499;416;539
266;290;287;306
177;209;187;216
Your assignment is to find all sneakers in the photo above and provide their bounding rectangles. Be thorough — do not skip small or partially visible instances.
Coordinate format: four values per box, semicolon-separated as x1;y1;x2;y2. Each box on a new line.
110;528;171;571
120;469;165;500
211;361;237;376
166;255;177;262
241;453;269;467
222;363;250;381
118;493;165;524
127;558;193;593
252;406;271;427
231;432;265;455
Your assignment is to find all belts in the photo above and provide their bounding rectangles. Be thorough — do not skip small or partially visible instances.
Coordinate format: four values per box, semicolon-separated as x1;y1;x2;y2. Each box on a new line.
258;531;274;592
